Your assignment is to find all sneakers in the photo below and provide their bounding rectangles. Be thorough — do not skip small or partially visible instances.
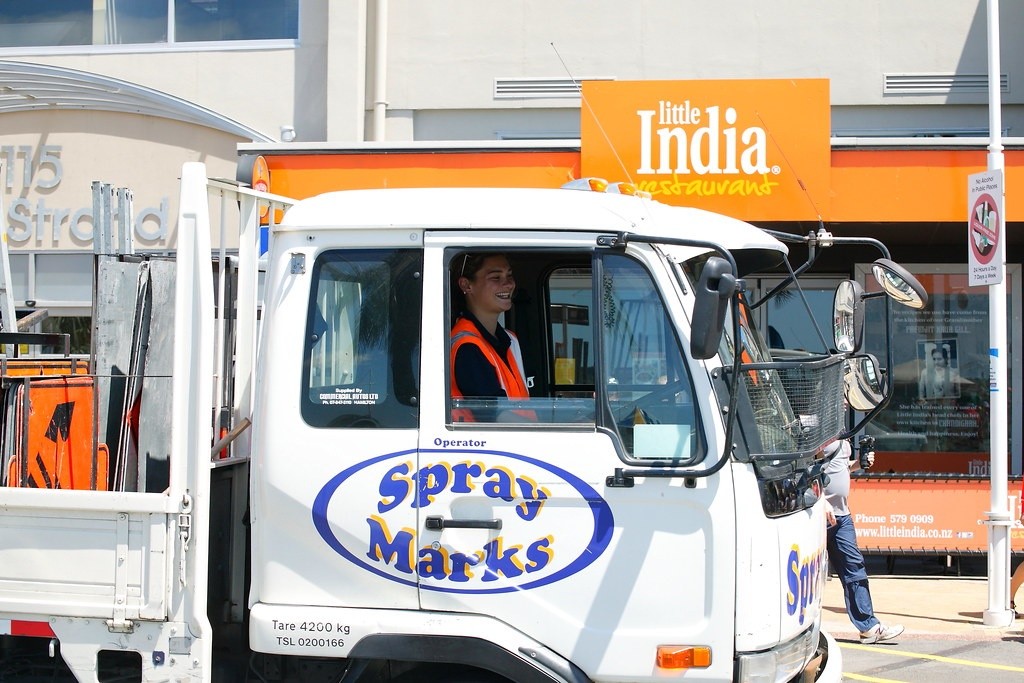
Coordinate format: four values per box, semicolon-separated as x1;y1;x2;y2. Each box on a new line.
860;623;904;644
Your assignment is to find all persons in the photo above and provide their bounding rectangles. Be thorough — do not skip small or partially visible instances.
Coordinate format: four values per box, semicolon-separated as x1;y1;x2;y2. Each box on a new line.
1010;477;1023;618
918;347;953;400
448;253;538;423
808;381;905;644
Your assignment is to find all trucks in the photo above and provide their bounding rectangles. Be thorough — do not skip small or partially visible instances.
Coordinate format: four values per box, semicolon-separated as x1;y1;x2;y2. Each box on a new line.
2;163;931;683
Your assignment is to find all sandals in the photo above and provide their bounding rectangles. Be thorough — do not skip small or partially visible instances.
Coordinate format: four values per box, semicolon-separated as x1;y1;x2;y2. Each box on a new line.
1010;601;1020;618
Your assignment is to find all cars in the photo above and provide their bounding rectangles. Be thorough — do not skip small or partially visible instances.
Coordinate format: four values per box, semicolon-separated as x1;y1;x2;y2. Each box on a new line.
863;418;929;452
